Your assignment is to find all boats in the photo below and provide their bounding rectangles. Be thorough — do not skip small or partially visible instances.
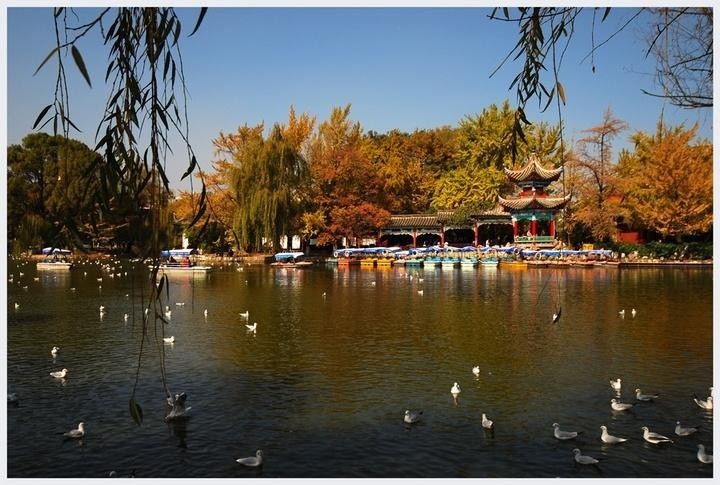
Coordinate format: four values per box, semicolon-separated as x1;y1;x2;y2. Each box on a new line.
334;247;612;267
154;271;213;283
36;247;74;270
147;248;213;270
337;265;608;272
269;252;313;268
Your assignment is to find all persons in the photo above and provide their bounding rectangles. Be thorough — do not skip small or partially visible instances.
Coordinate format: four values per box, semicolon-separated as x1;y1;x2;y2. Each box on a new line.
169;256;191;266
485;239;491;248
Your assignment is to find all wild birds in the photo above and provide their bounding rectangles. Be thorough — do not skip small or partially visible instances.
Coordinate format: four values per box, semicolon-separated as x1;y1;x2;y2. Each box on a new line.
551;423;584;440
166;392;186;406
599;379;713;464
51;346;59;354
8;392;17;401
245;322;258;330
99;303;250;320
163;335;175;343
62;421;90;438
450;382;461;394
50;367;70;380
403;409;423;423
481;412;494;430
236;448;264;468
472;365;481;375
571;448;599;465
